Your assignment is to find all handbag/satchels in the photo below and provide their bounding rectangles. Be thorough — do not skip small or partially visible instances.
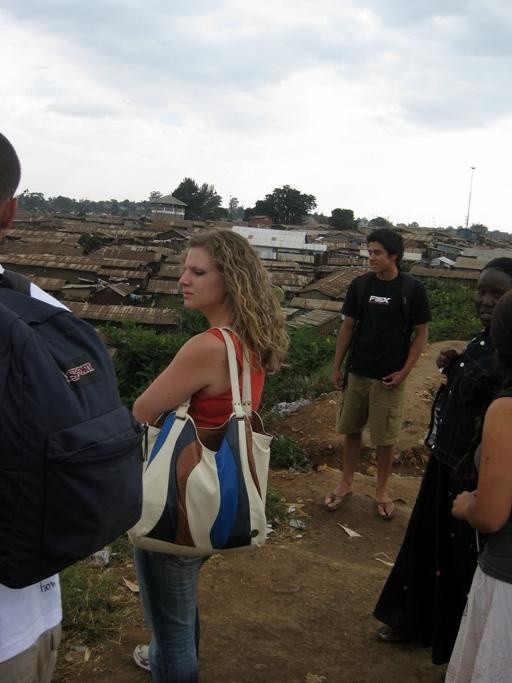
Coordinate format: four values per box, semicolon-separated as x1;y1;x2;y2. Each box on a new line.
124;328;274;555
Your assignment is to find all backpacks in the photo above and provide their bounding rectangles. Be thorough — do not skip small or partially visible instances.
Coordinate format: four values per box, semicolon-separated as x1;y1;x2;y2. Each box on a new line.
0;267;146;589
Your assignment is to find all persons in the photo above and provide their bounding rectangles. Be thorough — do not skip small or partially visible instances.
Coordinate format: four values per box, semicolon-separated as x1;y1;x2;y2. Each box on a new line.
369;255;512;666
322;228;430;520
0;133;76;682
441;280;512;683
120;230;291;682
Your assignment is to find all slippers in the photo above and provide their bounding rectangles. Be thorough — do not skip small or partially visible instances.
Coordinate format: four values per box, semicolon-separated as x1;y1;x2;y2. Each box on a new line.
375;498;396;520
322;488;351;512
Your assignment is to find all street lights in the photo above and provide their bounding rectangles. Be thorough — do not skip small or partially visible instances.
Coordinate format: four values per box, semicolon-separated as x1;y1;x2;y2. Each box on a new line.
466;165;479;227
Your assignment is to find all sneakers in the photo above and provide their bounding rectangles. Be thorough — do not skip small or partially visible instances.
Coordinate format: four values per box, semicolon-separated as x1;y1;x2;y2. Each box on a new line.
133;642;150;672
